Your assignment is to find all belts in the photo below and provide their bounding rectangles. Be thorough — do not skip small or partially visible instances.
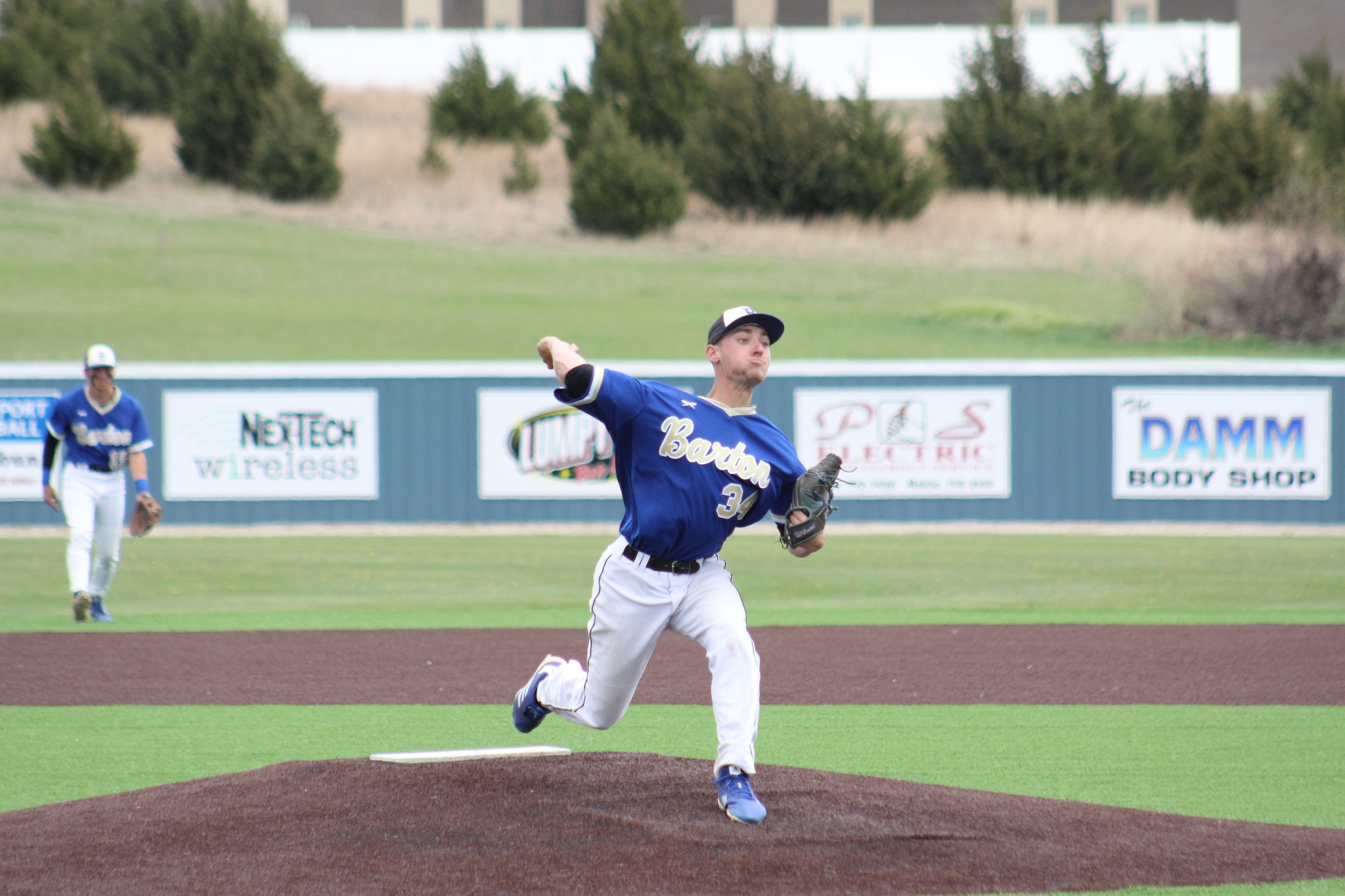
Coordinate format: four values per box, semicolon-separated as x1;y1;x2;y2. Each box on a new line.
622;545;700;575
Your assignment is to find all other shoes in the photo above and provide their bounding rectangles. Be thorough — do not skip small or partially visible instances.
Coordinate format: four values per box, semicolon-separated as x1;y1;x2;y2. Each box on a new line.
90;595;112;623
73;592;92;622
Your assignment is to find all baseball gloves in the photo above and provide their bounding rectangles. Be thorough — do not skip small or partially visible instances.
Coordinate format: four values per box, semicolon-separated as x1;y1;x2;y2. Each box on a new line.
129;491;164;538
783;453;843;549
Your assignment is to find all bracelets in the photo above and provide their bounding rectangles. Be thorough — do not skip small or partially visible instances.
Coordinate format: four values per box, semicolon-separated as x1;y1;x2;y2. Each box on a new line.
134;479;151;494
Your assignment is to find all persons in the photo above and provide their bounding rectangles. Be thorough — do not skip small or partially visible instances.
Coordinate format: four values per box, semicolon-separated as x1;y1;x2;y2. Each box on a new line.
41;342;163;626
512;306;825;827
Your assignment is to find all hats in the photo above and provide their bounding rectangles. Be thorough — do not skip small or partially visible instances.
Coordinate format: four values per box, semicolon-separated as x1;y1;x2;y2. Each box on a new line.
85;343;118;369
707;305;785;346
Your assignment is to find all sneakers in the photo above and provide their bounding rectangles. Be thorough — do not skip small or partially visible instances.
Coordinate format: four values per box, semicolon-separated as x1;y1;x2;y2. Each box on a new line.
511;654;567;735
715;765;768;826
